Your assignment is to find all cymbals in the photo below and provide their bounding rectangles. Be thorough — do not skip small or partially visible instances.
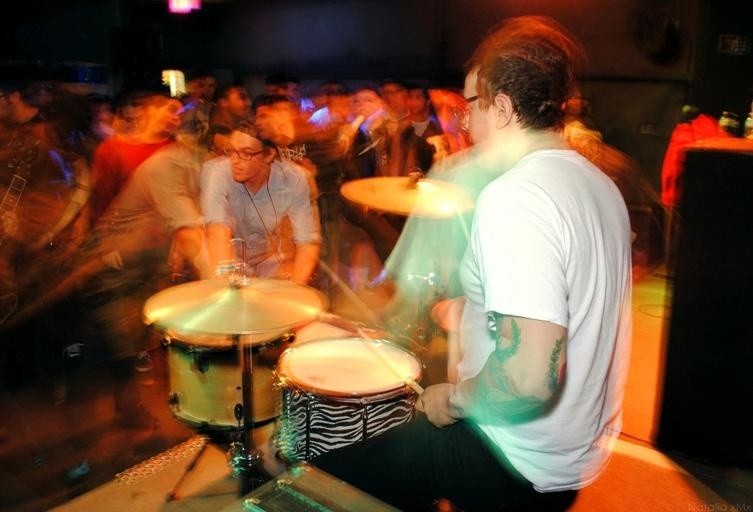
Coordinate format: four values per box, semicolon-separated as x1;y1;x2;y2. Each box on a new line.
142;276;327;334
341;176;472;217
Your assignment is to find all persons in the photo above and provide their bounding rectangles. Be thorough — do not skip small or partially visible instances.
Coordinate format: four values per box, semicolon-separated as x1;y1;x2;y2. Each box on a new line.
0;70;665;430
311;14;632;512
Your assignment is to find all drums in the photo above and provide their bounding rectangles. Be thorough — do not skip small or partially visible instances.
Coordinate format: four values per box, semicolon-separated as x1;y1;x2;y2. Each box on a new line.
161;327;302;429
274;336;425;469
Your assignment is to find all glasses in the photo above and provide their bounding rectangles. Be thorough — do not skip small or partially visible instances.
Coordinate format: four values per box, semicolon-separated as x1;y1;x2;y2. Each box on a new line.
453;91;490;130
221;143;268;163
121;114;144;124
